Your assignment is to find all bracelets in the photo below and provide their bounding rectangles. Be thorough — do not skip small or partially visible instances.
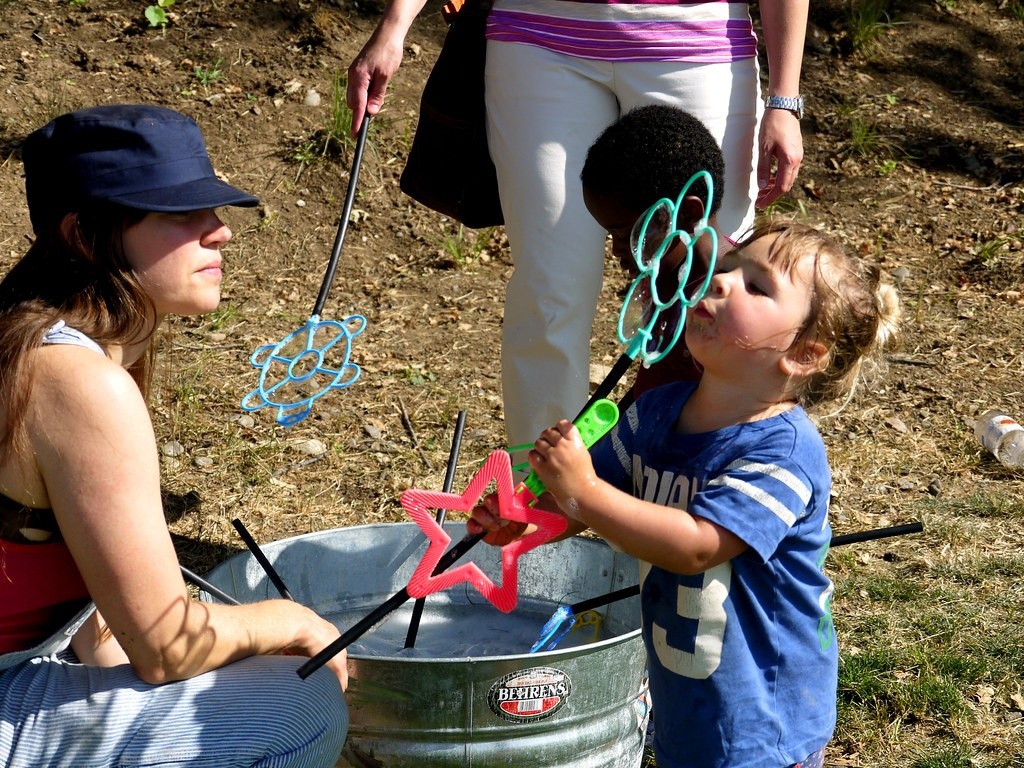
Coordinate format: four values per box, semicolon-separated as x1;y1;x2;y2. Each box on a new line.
764;95;805;119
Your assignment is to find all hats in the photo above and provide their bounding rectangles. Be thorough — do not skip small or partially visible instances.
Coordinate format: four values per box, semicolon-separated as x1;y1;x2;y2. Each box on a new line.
20;105;261;235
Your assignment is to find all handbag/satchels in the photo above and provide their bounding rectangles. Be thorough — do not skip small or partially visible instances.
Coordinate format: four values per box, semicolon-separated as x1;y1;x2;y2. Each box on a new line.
396;1;505;230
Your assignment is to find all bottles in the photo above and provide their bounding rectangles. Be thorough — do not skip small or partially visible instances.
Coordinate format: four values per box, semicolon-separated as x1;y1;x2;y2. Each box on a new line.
975;410;1024;471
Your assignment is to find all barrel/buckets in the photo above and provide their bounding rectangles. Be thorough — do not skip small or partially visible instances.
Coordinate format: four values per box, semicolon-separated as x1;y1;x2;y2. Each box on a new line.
197;522;652;768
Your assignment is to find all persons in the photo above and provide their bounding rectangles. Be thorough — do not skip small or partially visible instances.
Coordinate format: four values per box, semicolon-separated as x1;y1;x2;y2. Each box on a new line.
580;105;741;394
538;211;880;768
345;0;810;486
0;101;349;768
